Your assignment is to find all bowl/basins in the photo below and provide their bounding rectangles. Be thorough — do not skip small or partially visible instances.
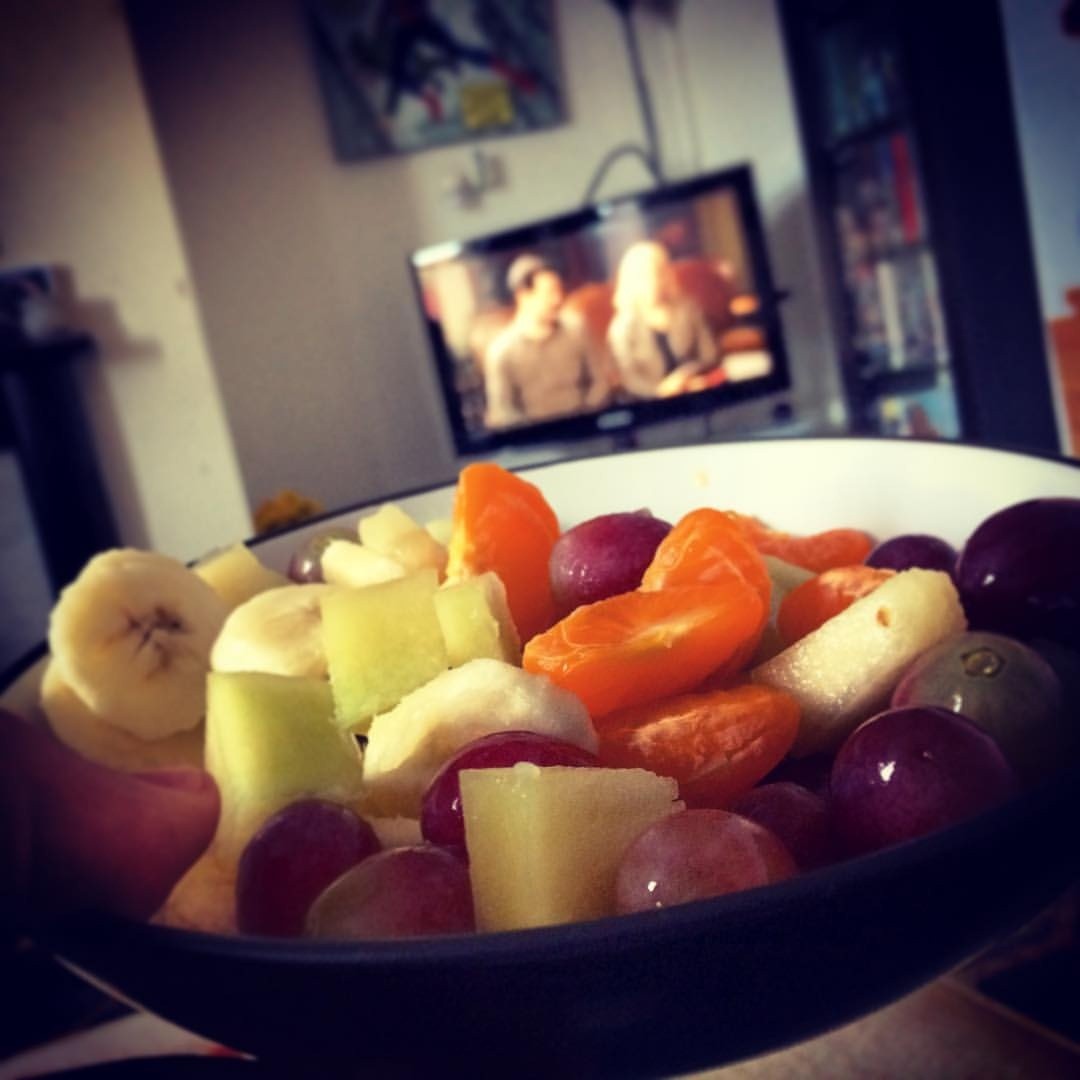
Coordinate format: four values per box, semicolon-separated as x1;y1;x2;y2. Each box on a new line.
0;438;1080;1080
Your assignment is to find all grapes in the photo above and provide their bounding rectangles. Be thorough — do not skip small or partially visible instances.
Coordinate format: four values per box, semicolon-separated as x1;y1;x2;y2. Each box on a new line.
228;495;1080;943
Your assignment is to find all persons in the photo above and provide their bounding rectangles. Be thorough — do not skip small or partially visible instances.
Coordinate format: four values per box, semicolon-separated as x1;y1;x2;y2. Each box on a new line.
606;240;723;399
484;253;622;428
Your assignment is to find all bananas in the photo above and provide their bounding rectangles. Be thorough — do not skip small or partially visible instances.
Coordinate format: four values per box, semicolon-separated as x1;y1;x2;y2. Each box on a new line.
37;543;968;938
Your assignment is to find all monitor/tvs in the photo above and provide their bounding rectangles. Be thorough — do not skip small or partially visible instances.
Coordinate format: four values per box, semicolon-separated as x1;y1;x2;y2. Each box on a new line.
405;157;794;460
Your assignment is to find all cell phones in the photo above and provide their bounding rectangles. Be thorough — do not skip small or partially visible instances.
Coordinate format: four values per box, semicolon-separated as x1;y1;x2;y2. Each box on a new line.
941;894;1080;1066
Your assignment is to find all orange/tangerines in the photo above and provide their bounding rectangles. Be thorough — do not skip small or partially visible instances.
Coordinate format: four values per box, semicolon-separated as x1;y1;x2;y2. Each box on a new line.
445;460;901;805
